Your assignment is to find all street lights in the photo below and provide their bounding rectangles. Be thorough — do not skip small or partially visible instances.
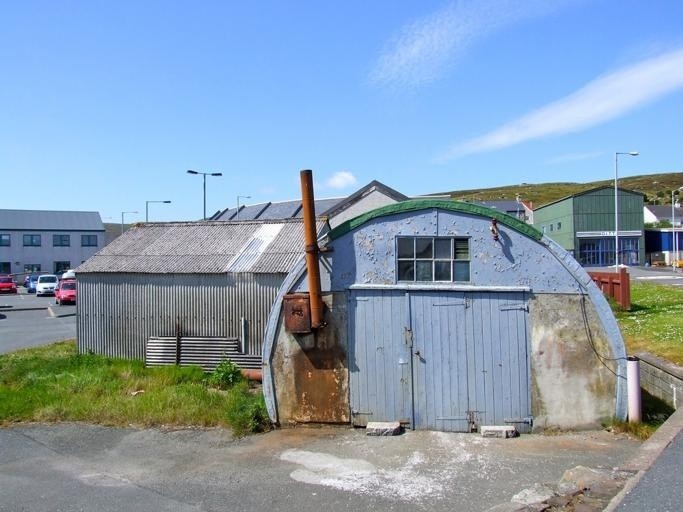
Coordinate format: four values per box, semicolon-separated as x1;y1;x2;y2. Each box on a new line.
146;201;171;222
237;195;252;220
672;187;683;272
187;170;223;220
615;151;641;272
122;211;138;234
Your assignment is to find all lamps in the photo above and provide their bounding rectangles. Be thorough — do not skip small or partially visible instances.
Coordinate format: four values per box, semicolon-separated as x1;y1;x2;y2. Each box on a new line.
491;215;501;247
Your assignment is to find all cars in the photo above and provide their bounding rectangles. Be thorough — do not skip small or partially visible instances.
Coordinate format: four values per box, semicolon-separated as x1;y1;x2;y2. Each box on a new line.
0;269;76;307
